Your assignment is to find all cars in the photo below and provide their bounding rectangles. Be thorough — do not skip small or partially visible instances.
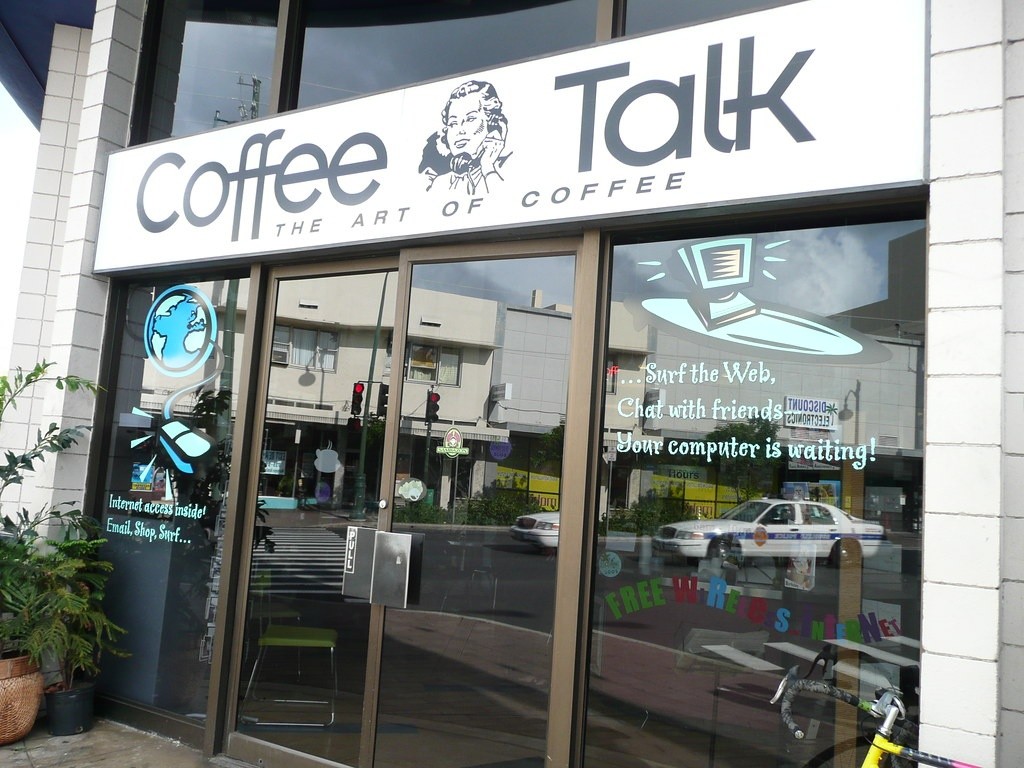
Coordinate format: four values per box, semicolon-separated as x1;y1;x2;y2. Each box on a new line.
650;498;884;571
508;509;561;549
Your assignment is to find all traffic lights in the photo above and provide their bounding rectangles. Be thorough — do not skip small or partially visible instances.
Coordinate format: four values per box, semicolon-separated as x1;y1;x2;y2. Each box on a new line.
352;420;361;431
428;392;440;422
352;382;365;415
380;384;389;415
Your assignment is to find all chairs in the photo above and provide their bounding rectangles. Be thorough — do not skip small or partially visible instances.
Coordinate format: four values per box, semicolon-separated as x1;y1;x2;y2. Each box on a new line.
237;564;339;729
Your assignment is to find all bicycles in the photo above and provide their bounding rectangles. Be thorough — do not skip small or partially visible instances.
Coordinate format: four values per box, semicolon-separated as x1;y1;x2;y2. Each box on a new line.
770;674;982;768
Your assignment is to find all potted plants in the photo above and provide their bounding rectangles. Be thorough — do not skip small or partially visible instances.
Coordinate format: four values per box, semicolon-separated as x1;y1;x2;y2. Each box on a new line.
0;358;112;744
28;539;133;736
